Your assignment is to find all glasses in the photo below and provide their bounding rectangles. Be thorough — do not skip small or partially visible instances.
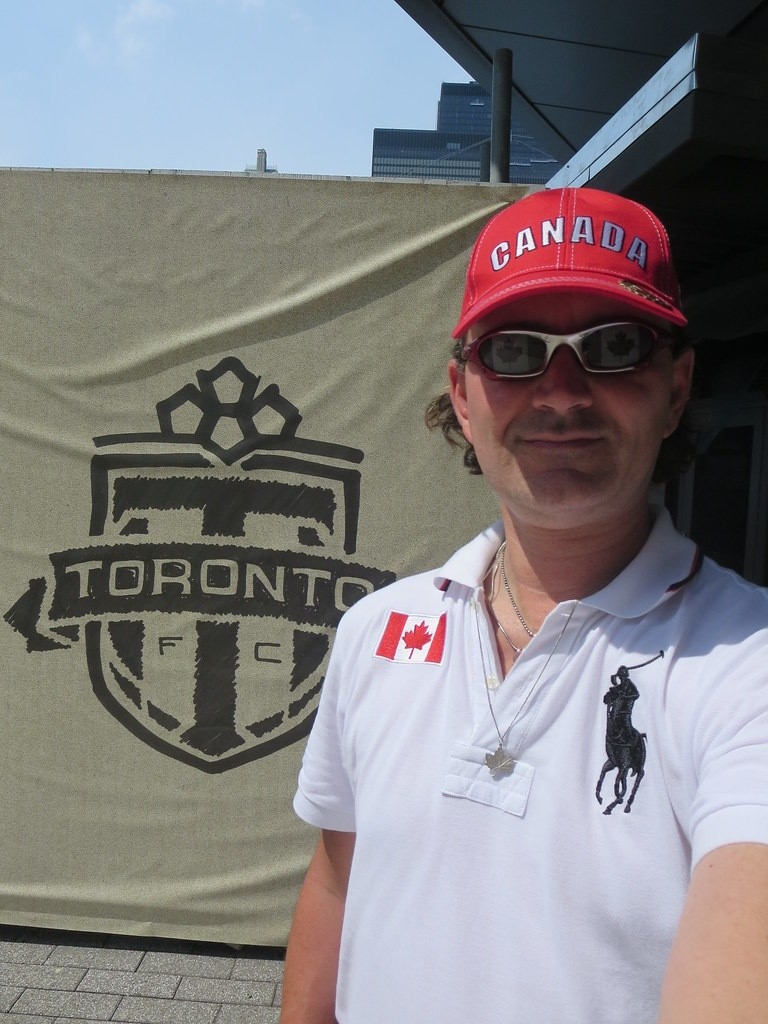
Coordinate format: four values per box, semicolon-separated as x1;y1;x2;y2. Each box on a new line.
460;314;674;383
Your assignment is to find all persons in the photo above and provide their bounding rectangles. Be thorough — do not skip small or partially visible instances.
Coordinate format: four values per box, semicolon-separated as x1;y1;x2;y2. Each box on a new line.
277;190;768;1024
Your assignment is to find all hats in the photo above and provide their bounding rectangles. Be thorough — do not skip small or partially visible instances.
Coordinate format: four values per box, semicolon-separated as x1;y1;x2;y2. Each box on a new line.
452;189;690;339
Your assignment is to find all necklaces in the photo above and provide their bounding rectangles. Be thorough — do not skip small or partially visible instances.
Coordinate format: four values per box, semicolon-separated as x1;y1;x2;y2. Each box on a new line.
484;544;534;664
471;562;608;779
